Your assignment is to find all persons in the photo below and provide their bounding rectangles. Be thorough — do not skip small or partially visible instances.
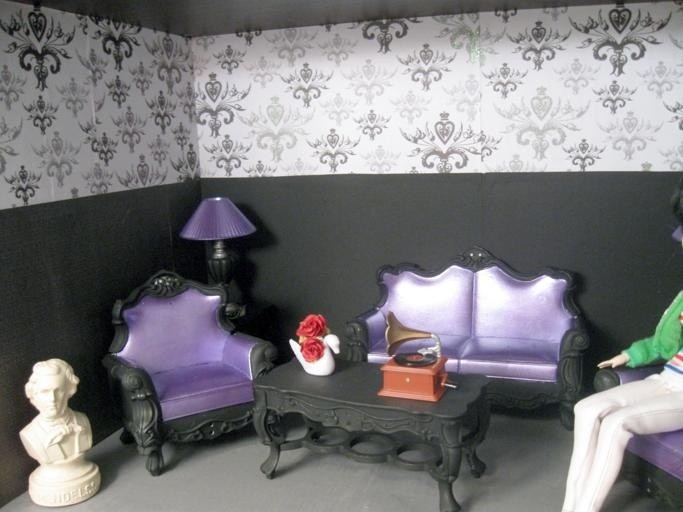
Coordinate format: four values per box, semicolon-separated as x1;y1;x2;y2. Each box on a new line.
19;358;92;468
559;181;683;512
